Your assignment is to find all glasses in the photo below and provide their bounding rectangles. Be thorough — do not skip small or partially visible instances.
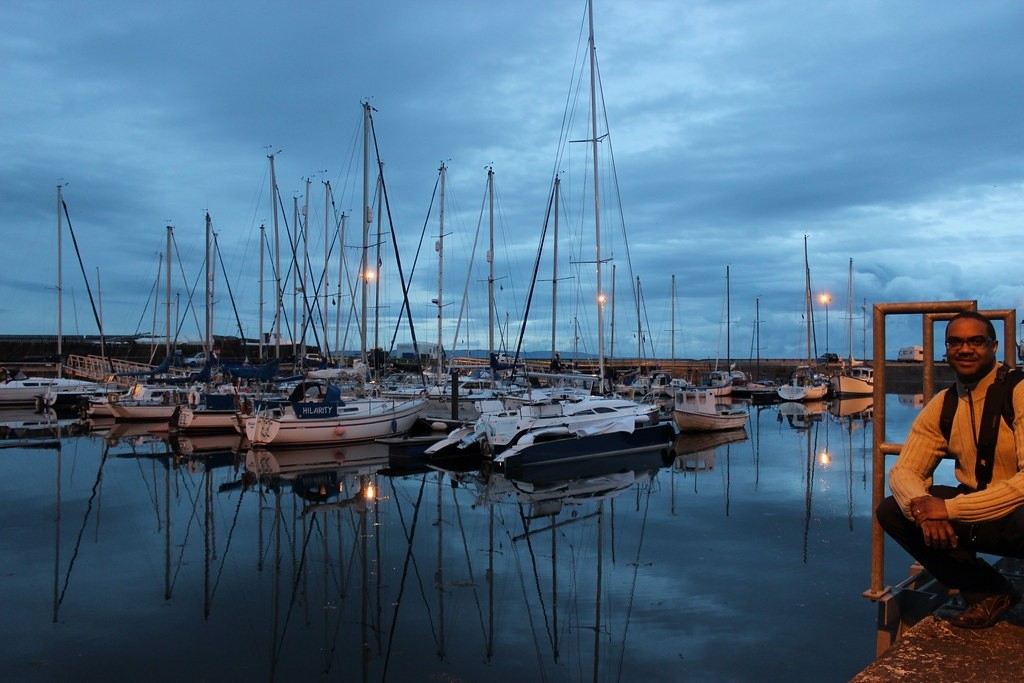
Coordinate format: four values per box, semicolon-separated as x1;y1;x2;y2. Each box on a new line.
945;336;993;349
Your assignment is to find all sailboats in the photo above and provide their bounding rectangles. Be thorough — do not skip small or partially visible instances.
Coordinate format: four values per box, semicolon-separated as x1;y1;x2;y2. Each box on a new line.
0;145;732;435
425;0;680;482
0;403;749;683
730;233;873;401
731;393;873;564
229;93;431;445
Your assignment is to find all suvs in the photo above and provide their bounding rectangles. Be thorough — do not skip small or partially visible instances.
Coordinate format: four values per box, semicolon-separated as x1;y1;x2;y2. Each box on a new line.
495;353;513;364
818;352;838;363
297;353;333;365
182;351;217;367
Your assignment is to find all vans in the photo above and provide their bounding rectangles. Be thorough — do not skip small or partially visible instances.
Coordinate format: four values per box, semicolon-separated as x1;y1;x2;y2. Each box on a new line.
897;346;923;363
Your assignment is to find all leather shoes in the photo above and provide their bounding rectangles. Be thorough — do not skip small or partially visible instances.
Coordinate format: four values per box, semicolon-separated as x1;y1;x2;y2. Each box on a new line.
953;581;1021;629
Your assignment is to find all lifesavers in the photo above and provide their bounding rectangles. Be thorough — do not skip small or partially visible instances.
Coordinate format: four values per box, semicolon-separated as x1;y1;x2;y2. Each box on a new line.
803;377;811;385
108;392;119;403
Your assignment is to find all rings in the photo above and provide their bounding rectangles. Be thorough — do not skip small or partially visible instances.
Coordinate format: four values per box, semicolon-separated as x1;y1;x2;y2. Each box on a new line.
916;509;921;513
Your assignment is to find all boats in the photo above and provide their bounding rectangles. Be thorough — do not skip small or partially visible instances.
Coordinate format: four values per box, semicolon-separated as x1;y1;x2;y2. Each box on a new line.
674;389;750;430
371;435;450;456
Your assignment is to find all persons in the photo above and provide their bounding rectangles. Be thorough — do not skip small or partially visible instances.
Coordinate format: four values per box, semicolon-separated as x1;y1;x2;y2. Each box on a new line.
875;311;1024;628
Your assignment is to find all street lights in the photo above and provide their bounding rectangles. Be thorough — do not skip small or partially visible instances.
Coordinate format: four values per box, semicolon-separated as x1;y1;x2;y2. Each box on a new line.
820;295;831;353
